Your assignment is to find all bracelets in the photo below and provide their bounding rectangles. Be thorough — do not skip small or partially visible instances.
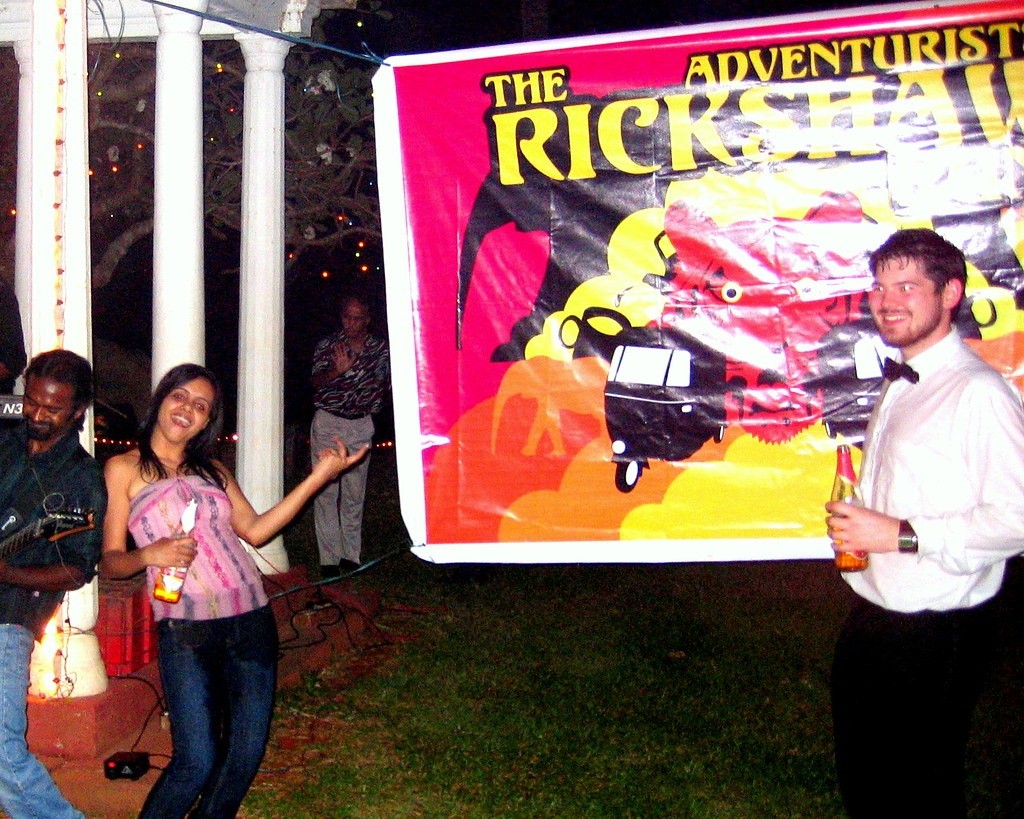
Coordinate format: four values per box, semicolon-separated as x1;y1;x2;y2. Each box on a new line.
898;520;918;553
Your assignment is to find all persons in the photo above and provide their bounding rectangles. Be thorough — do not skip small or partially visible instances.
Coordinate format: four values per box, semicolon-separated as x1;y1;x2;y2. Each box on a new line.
0;281;27;395
309;297;391;579
101;364;366;819
0;349;108;819
825;228;1024;819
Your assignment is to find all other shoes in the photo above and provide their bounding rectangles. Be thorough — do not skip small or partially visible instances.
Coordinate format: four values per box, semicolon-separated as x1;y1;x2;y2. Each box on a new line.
340;559;367;574
319;565;335;578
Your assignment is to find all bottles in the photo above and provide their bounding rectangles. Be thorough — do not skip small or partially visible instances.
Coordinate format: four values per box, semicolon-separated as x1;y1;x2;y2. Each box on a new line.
153;498;200;605
831;445;869;572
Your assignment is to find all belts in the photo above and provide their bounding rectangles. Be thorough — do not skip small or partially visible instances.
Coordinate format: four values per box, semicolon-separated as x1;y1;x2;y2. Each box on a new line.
334;411;367;420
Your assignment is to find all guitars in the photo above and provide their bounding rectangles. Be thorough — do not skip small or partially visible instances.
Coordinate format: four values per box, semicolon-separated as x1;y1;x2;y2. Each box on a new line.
0;507;96;562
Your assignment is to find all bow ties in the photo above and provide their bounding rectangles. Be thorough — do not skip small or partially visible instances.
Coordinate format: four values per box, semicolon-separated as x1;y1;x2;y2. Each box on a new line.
883;357;919;384
